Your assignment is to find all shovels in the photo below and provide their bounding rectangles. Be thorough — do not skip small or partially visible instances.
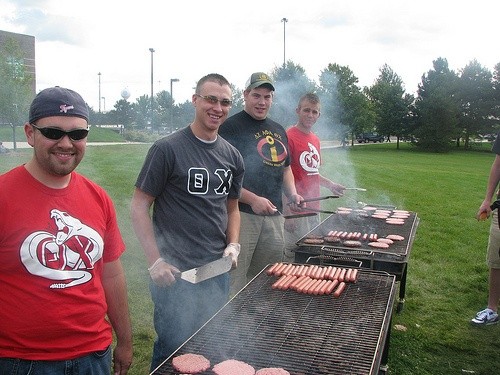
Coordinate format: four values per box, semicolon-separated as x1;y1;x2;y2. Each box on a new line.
171;255;232;284
272;208;317;219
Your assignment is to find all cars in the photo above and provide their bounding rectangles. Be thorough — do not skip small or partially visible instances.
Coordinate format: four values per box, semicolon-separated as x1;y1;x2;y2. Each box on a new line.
356;132;384;143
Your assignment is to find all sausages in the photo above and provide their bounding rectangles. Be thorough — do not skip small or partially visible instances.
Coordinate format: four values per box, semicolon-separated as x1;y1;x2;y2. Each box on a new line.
266;263;358;297
328;230;378;241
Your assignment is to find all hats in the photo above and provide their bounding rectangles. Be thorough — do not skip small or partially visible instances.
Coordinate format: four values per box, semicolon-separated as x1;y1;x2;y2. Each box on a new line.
243;71;276;92
28;85;90;123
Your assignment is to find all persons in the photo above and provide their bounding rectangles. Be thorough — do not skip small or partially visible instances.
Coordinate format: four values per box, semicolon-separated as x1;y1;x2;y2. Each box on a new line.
127;73;246;374
219;72;306;304
283;93;346;265
471;135;500;326
0;85;134;375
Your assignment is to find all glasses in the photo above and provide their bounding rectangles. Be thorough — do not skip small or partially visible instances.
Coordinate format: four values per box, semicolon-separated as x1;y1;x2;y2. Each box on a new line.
195;93;234;108
30;122;90;142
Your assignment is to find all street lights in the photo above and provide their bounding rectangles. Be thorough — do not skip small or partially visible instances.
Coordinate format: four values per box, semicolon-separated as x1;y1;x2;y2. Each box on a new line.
98;72;101;127
149;47;156;130
281;17;289;68
101;96;105;113
170;78;180;133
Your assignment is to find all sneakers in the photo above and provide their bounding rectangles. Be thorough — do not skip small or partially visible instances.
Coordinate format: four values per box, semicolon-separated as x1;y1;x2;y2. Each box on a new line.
472;308;500;326
